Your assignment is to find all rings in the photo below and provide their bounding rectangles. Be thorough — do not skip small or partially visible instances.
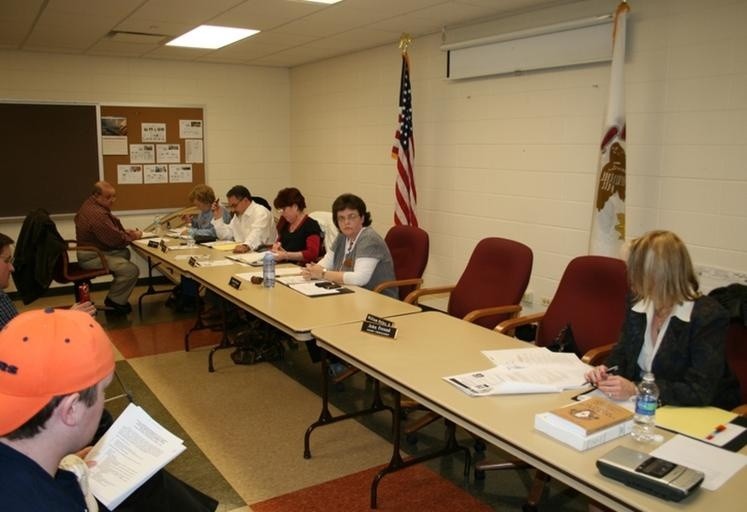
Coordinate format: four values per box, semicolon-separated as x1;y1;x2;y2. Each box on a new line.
606;391;614;400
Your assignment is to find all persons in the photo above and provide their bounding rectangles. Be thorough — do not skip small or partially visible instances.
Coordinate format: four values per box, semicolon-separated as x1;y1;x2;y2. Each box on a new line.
73;180;144;312
234;189;326;349
583;228;745;413
2;234;115;447
0;305;221;511
195;184;281;320
304;194;399;377
166;184;232;307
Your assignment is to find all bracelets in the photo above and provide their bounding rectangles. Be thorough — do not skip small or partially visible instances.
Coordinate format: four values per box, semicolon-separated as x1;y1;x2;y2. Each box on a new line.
283;250;290;261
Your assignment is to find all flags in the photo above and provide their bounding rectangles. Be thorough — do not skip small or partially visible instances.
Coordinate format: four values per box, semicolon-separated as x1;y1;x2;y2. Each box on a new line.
390;47;423;231
581;2;631;265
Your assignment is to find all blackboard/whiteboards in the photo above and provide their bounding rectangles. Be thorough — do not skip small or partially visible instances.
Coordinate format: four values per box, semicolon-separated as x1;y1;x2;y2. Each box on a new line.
1;100;104;221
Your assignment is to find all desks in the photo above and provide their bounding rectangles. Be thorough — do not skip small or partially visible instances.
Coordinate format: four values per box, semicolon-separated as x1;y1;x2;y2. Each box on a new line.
189;260;423;461
130;232;252;352
311;311;746;511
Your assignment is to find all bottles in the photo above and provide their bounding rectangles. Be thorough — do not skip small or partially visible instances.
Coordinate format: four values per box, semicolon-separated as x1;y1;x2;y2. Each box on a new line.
76;280;89;305
263;251;276;289
631;375;659;442
186;223;195;252
153;215;162;238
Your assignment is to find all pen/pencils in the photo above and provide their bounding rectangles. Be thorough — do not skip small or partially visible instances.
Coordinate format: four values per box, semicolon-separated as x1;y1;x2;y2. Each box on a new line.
580;365;619;387
212;198;220;212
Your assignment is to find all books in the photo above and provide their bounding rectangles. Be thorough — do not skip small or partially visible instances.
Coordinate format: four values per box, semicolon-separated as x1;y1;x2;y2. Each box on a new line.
534;395;633;452
548;396;634;437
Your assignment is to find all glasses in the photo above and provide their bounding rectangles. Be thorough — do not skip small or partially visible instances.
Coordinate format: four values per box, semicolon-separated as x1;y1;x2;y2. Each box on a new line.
336;213;361;222
231;194;248;209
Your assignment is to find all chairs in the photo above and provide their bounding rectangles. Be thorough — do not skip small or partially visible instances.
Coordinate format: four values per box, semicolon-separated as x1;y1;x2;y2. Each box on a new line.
54;207;113;303
493;254;628;368
374;223;430;304
404;237;534;328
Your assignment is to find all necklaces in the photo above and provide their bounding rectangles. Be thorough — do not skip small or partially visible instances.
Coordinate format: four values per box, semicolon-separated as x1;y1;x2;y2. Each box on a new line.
650;308;666;338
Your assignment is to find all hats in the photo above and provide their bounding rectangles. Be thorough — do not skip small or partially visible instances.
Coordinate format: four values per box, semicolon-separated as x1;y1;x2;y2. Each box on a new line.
0;305;117;439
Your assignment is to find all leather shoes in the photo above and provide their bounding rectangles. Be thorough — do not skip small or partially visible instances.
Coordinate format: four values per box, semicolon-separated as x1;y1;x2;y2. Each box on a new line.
104;296;132;313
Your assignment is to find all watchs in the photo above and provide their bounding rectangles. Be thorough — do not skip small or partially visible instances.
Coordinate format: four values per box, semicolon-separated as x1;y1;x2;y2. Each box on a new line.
320;268;327;279
